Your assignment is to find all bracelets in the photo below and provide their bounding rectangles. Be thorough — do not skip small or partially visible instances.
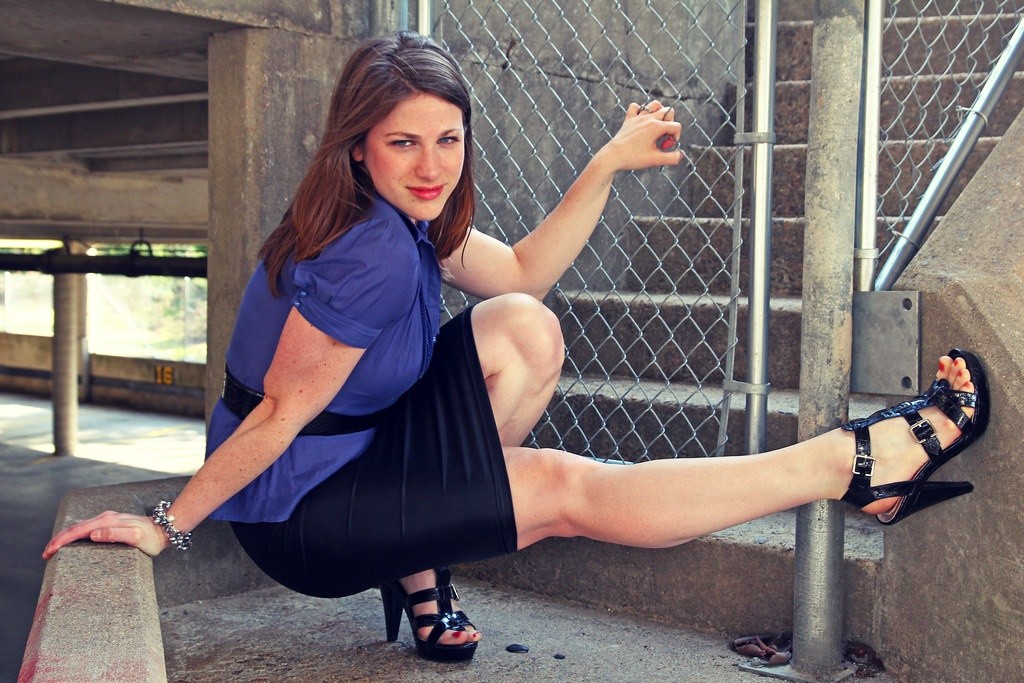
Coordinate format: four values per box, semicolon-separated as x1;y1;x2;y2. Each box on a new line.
152;500;193;550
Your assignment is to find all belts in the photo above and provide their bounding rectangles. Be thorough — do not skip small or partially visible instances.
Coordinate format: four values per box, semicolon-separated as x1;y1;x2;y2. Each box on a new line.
224;364;391;436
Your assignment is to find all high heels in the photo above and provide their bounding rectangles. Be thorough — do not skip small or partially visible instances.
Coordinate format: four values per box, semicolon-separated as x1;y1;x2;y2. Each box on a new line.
841;348;990;526
381;568;481;662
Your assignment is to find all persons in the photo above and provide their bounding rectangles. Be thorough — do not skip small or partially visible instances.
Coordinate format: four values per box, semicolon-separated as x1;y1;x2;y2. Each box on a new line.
44;32;990;660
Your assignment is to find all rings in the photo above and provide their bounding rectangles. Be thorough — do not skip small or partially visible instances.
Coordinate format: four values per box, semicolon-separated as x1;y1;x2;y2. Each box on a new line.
637;103;653;114
